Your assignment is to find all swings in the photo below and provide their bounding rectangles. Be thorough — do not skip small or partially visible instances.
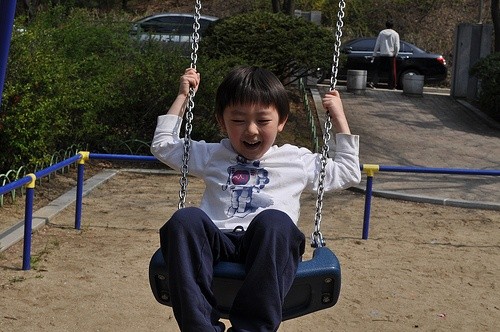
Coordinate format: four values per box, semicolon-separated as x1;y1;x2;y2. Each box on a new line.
149;0;344;321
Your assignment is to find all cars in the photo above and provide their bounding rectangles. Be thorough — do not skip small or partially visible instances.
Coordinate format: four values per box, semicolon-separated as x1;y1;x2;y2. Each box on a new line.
125;13;217;43
315;36;447;91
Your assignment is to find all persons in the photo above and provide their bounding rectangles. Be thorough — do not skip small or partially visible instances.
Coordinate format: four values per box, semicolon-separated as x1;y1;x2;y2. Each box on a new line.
150;66;361;332
368;18;400;90
